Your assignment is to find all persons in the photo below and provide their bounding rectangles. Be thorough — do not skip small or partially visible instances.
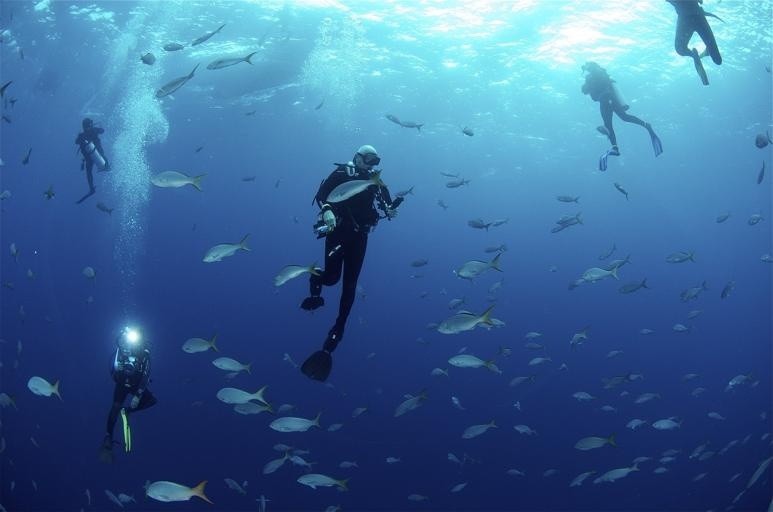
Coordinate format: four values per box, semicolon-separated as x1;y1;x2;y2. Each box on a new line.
667;0;722;84
581;61;662;173
104;326;159;458
300;144;405;382
74;118;111;203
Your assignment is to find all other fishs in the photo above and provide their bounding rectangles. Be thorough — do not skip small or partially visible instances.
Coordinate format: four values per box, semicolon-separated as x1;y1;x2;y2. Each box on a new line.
0;9;773;512
